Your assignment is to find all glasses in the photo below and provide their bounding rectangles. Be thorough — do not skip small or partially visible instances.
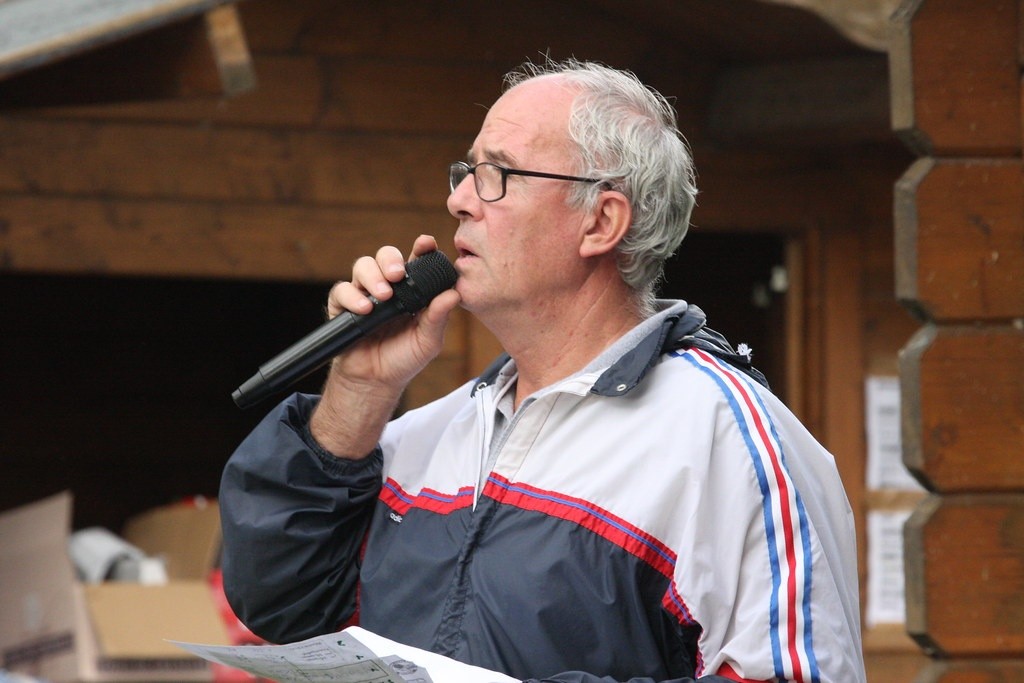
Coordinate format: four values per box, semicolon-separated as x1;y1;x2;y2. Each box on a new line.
448;159;617;201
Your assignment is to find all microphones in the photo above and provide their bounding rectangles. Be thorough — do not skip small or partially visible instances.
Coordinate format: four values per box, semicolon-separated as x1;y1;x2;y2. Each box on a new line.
232;249;460;411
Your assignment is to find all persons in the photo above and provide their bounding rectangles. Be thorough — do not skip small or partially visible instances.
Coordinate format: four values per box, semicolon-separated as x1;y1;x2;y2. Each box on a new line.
218;63;868;683
